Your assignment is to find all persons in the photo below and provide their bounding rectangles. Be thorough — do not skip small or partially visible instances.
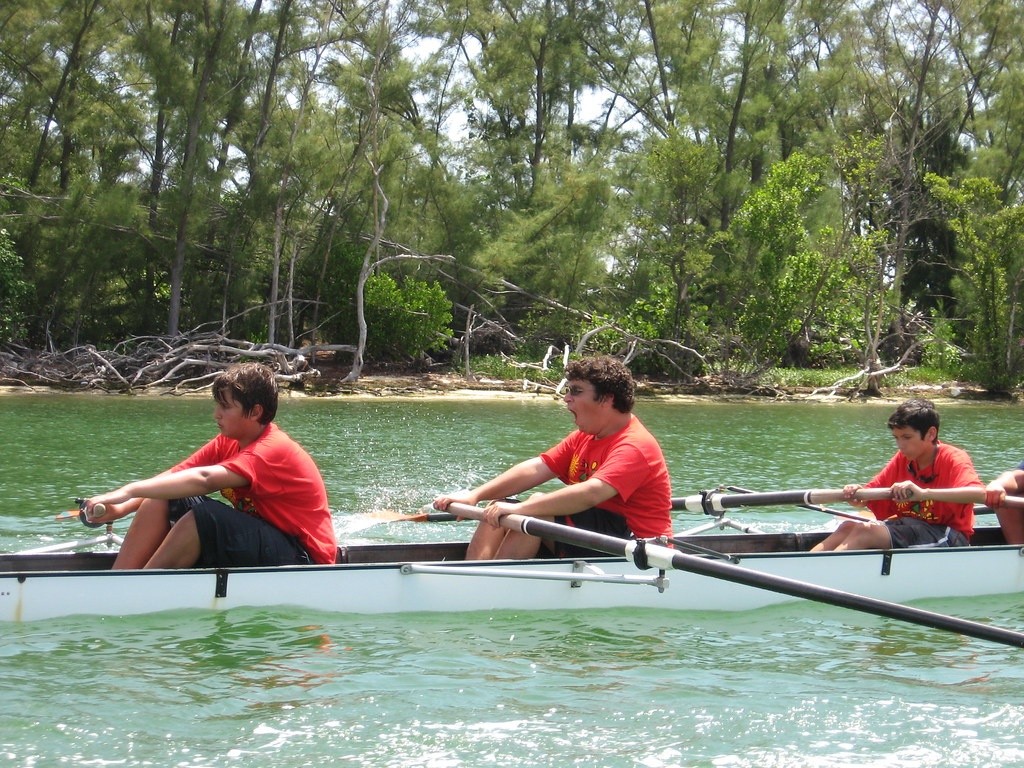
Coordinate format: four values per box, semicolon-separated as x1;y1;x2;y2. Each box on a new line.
985;458;1024;545
810;398;987;552
86;363;337;570
433;356;674;560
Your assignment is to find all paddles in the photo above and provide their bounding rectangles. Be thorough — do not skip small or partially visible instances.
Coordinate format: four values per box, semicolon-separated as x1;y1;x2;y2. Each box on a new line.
431;496;1023;647
974;491;1024;509
55;502;107;520
388;486;914;523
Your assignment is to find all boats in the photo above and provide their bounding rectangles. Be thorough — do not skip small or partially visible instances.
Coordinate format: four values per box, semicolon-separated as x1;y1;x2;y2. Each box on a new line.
0;525;1024;622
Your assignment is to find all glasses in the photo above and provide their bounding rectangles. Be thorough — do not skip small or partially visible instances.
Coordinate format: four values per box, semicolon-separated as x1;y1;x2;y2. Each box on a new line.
566;385;597;396
